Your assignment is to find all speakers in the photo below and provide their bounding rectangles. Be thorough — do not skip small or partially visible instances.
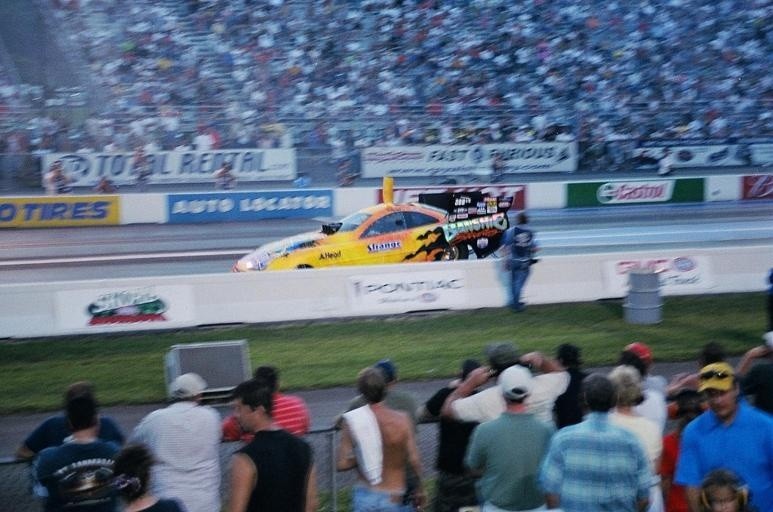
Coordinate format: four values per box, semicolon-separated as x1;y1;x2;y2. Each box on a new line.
164;339;253;402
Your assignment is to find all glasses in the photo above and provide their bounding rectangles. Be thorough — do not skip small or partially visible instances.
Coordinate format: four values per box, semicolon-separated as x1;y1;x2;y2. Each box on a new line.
699;369;735;380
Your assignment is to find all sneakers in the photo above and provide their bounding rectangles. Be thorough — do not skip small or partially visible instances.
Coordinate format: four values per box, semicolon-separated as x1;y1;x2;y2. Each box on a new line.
512;301;527;312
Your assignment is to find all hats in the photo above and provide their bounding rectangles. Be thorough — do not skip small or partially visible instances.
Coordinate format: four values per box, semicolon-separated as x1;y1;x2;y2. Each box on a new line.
369;358;398;386
620;340;653;368
496;364;535;402
695;361;735;394
166;372;208;401
484;338;520;371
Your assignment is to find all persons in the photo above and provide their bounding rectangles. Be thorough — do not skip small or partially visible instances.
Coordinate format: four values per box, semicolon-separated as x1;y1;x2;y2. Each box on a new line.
489;212;540;313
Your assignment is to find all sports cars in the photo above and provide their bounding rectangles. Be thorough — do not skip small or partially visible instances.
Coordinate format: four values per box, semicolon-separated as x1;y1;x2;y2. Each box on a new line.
229;176;520;269
